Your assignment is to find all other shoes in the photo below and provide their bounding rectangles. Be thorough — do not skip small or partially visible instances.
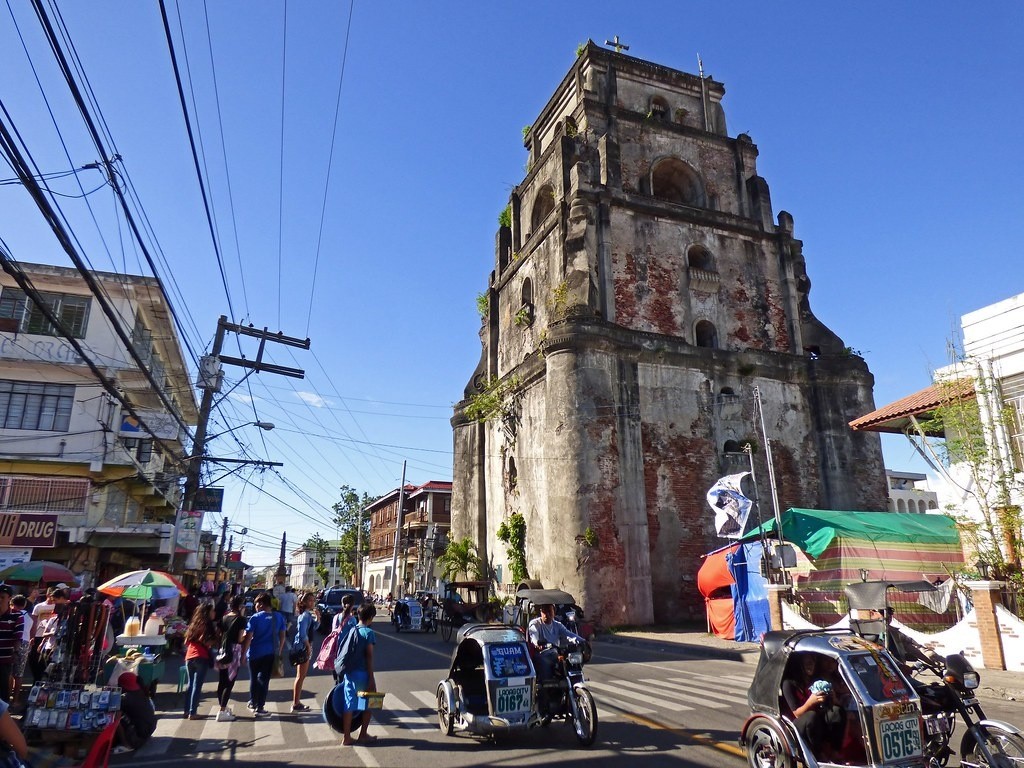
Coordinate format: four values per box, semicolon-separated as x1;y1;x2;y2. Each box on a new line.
189;714;204;720
184;712;189;718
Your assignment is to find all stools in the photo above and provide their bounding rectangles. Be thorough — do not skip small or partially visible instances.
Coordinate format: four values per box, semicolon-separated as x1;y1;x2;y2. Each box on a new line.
176;666;187;696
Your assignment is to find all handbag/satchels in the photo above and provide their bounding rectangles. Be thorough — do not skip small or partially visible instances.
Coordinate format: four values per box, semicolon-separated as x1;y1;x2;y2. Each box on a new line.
823;704;843;729
289;642;308;666
216;633;230;664
271;655;284;678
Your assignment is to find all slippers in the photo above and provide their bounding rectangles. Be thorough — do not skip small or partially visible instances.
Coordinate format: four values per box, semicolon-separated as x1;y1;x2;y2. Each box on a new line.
111;745;134;755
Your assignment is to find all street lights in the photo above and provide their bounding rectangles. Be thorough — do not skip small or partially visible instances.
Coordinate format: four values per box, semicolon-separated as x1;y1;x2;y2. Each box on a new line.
161;418;275;655
214;516;248;594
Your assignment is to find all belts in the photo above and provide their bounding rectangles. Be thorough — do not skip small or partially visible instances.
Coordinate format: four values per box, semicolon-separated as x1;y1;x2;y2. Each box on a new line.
47;601;108;684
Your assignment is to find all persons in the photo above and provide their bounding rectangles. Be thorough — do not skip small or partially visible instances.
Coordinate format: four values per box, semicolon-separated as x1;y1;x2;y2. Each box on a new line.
215;598;248;721
184;583;252;620
342;602;379;747
774;651;884;767
31;581;138;683
291;593;321;711
331;596;356;684
370;591;394;615
0;699;29;768
274;584;310;639
0;584;40;709
876;604;935;687
529;603;587;679
423;594;443;610
183;603;215;720
241;593;286;716
110;671;157;753
447;588;467;604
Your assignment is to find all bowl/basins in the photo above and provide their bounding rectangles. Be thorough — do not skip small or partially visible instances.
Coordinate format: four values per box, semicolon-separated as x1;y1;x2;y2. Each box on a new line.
322;684;362;734
332;682;361;719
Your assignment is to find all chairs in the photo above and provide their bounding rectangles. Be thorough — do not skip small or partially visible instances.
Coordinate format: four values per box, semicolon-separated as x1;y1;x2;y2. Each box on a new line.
455;668;487;708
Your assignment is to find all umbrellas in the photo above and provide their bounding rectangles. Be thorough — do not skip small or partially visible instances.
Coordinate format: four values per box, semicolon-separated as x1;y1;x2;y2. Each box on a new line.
0;559;77;591
97;568;188;634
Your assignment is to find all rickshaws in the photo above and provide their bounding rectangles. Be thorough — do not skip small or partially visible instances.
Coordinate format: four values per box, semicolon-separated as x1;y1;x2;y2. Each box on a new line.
736;580;1024;768
436;588;599;746
390;590;444;634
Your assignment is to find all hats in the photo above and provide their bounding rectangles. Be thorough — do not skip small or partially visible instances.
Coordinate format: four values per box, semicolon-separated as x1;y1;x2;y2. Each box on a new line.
118;671;140;691
56;583;70;589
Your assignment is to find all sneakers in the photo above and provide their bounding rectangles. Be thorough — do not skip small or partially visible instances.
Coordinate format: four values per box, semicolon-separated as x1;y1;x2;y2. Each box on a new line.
290;702;310;712
216;712;235;721
255;707;271;717
247;701;253;708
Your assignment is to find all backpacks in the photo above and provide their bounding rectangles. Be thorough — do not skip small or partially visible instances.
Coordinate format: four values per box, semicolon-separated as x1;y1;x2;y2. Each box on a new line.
333;625;367;675
313;615;350;672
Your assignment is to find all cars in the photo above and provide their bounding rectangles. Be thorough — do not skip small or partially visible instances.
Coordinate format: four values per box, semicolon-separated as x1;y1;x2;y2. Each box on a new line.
316;585;370;636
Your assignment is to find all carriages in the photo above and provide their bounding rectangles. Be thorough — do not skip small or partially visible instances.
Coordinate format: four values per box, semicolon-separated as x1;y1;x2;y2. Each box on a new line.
440;579;504;644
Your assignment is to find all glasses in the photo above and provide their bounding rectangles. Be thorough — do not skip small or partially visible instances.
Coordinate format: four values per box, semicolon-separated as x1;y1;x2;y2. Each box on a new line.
875;609;893;615
